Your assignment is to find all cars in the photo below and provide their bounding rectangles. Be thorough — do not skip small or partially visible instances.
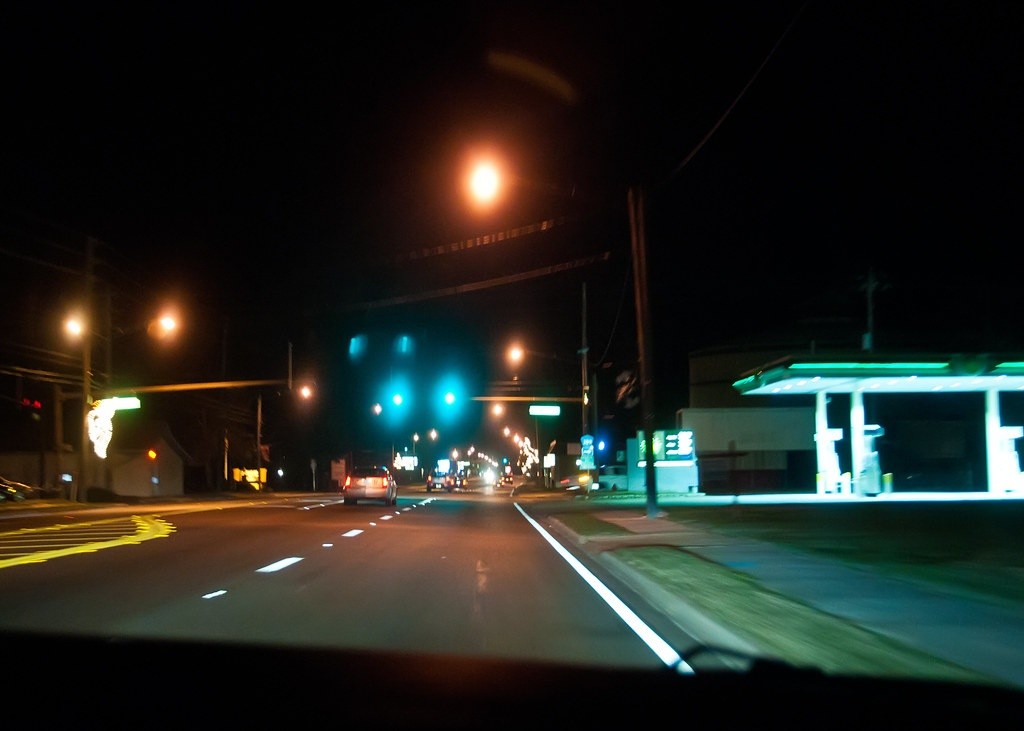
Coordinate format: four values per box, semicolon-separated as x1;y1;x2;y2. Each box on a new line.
426;467;468;493
493;473;513;490
343;464;399;506
0;477;33;503
